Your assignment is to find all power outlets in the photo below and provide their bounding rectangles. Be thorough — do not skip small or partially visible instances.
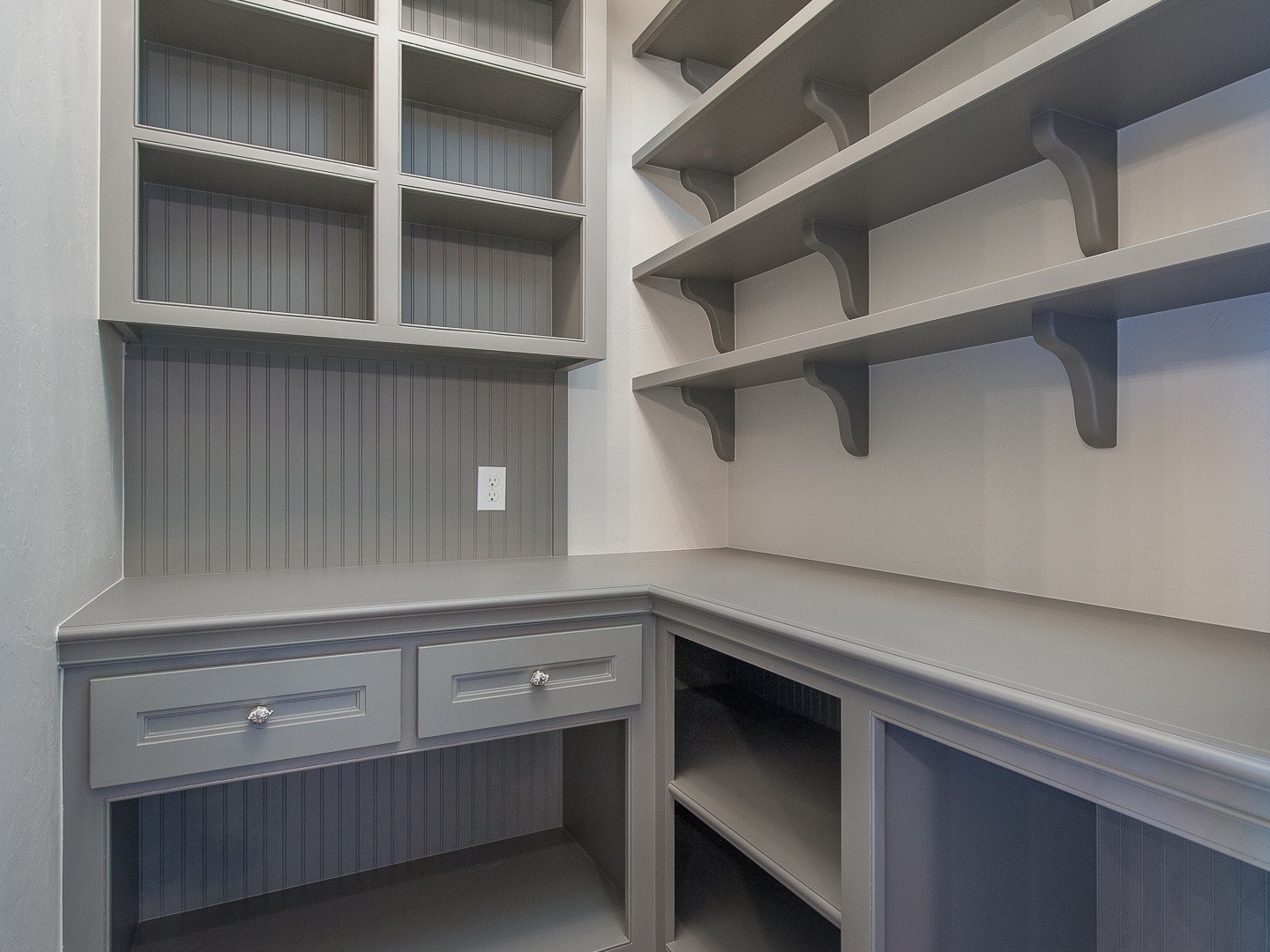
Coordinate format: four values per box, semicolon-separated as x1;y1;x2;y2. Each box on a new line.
477;466;506;511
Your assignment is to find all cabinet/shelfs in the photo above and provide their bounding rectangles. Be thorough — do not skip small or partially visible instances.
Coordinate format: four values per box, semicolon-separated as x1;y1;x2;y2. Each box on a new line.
57;543;1270;951
632;0;1269;463
100;0;607;376
